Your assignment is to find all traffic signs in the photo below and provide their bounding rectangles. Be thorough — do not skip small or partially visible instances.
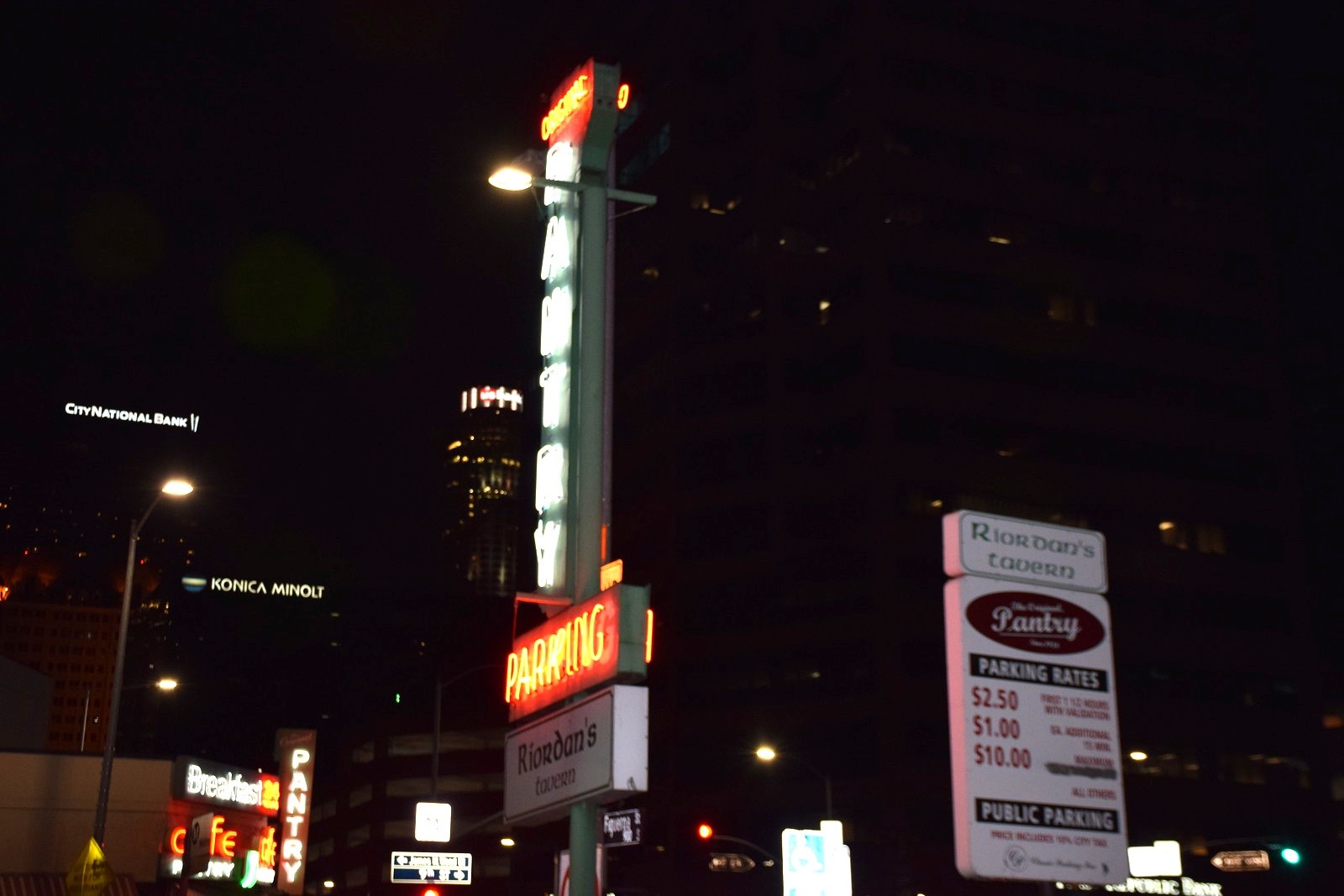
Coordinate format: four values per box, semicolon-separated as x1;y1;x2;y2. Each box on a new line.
391;851;472;885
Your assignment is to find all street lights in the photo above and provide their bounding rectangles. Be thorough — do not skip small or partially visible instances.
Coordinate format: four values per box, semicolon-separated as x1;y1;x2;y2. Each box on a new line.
756;745;833;820
484;166;658;896
93;478;193;852
696;824;782;868
